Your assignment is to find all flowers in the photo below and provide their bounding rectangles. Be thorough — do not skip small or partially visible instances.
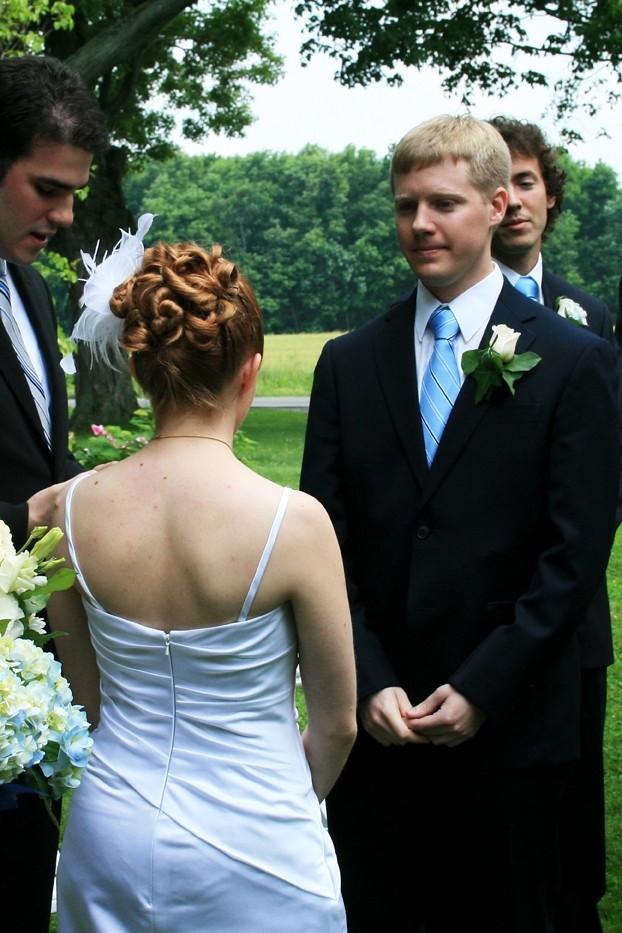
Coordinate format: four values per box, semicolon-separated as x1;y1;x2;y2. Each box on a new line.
557;296;589;327
0;517;94;791
63;208;155;373
462;323;542;403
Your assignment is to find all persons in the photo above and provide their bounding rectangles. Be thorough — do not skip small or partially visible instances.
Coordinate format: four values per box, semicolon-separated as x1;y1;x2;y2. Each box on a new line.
299;118;622;933
0;55;117;933
44;243;358;933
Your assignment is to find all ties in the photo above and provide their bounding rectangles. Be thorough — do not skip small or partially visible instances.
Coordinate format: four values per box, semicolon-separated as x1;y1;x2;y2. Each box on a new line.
513;276;538;303
0;274;54;454
418;308;461;471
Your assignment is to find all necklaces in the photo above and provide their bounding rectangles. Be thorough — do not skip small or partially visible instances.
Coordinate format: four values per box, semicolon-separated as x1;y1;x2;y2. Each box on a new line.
148;436;232;449
